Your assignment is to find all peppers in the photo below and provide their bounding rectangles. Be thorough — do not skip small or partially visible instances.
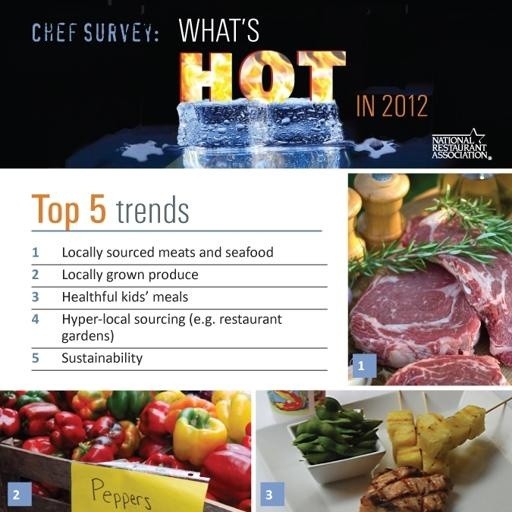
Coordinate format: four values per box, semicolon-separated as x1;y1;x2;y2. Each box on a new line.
2;391;252;512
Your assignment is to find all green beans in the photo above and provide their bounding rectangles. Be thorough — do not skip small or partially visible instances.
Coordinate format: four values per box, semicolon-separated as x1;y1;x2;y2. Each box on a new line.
292;398;384;465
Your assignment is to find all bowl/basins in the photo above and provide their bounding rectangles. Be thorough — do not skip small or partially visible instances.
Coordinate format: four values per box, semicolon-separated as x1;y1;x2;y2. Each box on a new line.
286;408;387;486
